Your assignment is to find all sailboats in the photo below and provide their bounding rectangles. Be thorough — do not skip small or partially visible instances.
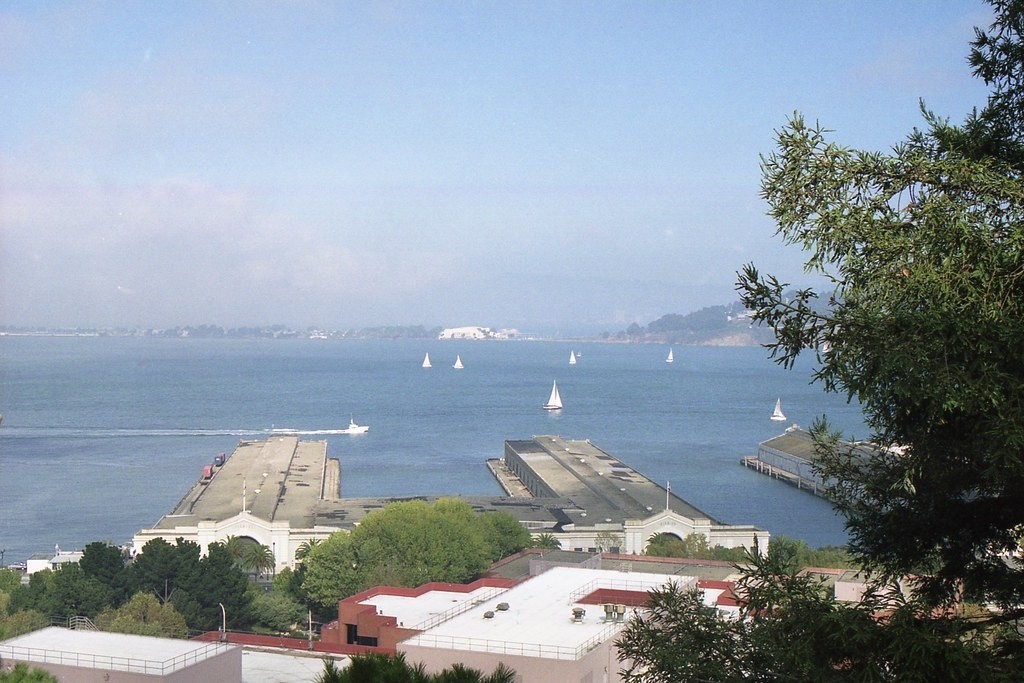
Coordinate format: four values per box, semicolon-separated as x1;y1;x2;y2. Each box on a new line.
543;378;564;410
665;348;675;363
568;349;577;365
421;352;432;368
768;398;788;422
452;354;465;369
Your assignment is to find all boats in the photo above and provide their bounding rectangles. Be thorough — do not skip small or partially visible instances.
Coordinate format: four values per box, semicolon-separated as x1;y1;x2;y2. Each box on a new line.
347;418;370;433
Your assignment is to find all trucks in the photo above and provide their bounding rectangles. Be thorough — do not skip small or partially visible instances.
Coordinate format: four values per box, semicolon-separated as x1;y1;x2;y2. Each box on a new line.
214;452;225;467
203;463;214;479
